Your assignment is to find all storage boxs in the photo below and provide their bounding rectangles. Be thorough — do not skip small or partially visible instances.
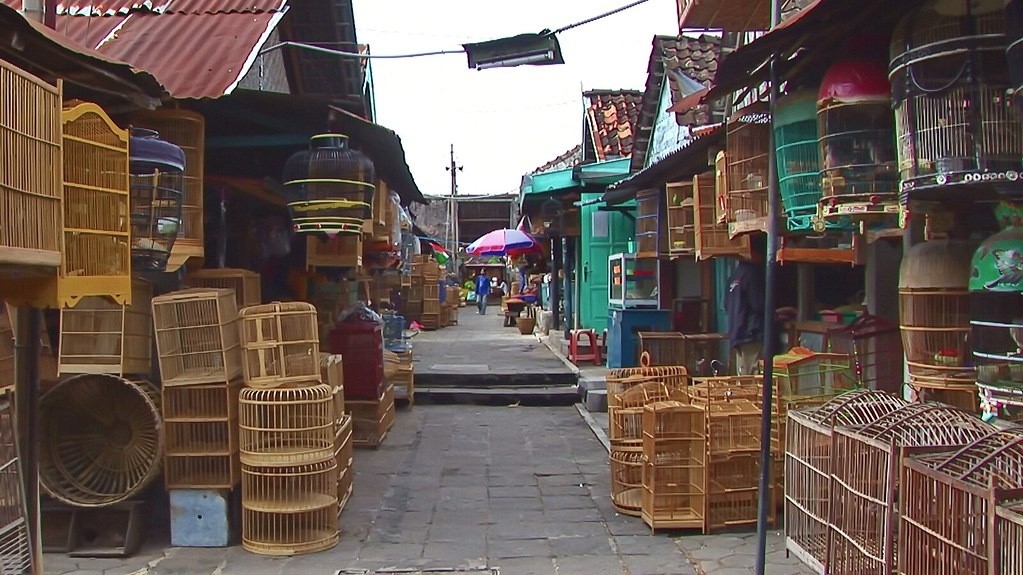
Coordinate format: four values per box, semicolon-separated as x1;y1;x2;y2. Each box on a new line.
64;500;150;558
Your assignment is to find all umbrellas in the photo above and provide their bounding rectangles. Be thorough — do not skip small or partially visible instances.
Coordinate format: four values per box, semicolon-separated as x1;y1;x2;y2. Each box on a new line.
465;227;534;281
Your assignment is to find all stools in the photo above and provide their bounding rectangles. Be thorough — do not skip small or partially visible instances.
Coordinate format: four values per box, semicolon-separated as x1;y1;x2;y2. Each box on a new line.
503;311;519;327
568;329;601;366
601;328;608;363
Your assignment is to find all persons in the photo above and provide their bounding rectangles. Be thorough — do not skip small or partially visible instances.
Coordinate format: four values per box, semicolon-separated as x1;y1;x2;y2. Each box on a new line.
723;238;779;375
497;279;509;296
475;268;491;315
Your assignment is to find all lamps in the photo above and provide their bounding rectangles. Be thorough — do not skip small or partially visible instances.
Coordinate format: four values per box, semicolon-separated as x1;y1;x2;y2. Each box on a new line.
461;30;563;70
